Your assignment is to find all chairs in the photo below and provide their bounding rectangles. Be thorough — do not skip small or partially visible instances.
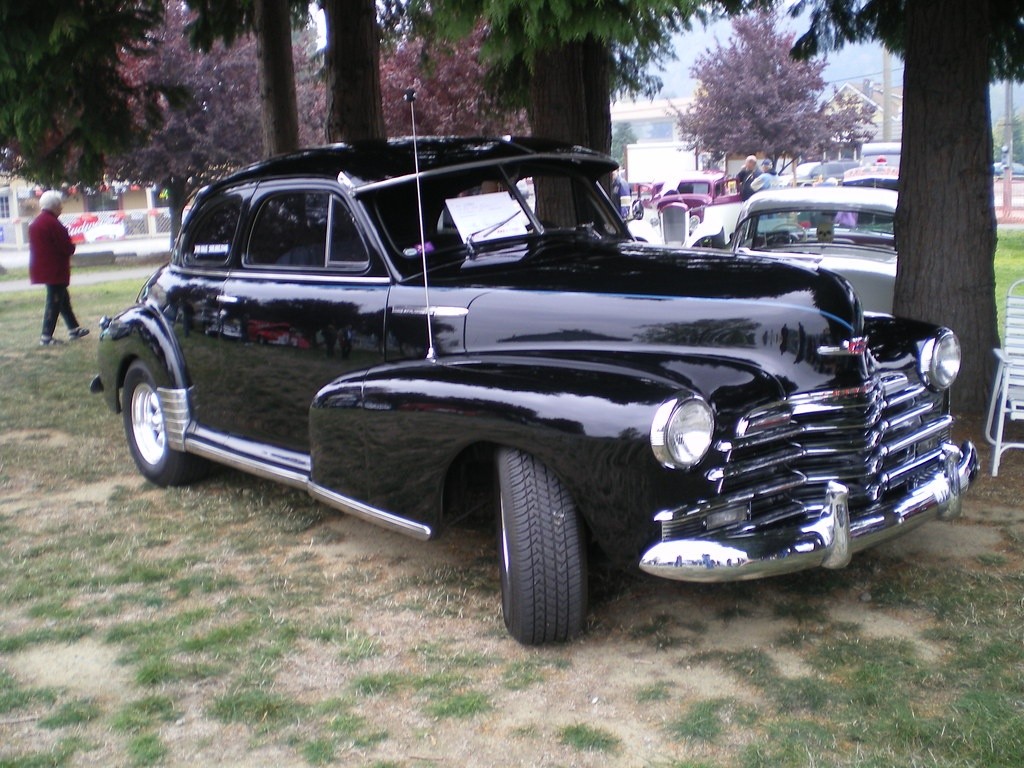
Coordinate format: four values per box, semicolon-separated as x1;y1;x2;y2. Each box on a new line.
274;242;324;266
986;279;1024;477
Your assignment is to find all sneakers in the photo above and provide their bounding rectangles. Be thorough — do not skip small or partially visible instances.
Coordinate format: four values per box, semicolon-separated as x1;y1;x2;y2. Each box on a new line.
40;336;63;347
69;329;89;340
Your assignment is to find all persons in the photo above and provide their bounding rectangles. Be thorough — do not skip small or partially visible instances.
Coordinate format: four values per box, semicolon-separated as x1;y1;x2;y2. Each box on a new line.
610;167;629;227
736;155;779;202
442;180;505;236
28;190;90;345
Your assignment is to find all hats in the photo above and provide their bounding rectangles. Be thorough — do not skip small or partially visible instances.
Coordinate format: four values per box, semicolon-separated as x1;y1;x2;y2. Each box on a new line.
761;159;771;166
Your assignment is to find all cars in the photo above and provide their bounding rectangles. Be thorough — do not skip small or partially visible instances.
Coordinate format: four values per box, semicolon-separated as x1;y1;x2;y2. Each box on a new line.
730;186;898;318
87;133;980;649
627;170;744;249
774;160;858;187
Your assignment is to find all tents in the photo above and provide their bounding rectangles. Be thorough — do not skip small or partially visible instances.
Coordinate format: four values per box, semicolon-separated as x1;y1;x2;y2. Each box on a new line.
793;158;899;182
